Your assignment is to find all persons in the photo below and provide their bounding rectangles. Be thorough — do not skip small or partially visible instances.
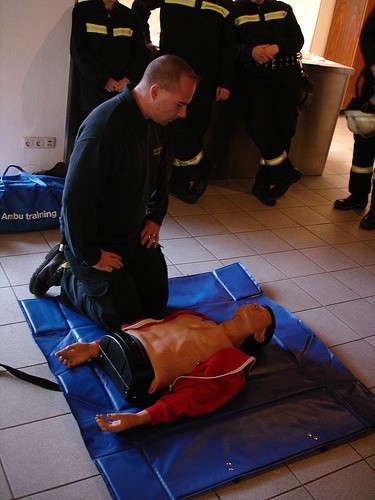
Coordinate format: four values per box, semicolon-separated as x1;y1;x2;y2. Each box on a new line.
129;0;236;202
56;300;276;432
231;0;308;206
333;9;375;230
70;0;145;144
29;54;198;327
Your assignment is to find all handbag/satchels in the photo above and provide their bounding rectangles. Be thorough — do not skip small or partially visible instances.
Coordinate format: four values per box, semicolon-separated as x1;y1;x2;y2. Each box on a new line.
0;164;64;233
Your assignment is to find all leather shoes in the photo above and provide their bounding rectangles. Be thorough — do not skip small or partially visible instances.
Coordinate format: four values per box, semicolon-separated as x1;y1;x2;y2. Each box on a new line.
28;243;67;297
359;212;375;229
335;190;370;211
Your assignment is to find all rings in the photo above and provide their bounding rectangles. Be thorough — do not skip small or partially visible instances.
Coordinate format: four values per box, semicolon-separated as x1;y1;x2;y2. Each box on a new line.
151;233;156;237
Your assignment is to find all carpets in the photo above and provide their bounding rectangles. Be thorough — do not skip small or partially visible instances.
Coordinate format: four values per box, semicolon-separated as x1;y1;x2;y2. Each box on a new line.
14;260;375;500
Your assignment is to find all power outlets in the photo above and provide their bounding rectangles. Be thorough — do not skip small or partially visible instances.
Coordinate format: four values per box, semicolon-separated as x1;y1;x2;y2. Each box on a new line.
22;137;56;149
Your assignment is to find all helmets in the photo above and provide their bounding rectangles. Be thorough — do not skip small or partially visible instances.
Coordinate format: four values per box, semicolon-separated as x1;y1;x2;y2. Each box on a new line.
344;109;375;138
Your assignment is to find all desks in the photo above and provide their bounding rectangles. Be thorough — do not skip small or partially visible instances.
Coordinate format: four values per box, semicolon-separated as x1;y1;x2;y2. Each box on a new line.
303;51;355;76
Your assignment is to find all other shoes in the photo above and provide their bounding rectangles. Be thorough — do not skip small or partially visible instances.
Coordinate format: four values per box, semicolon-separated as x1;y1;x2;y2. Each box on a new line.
272;169;303;200
251;180;276;207
